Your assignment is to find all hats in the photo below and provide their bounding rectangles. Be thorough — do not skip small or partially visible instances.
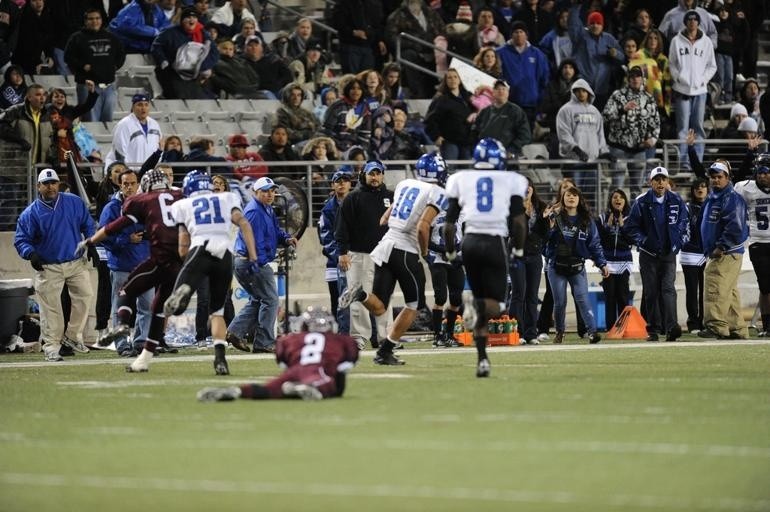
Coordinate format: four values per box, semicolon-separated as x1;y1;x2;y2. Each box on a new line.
456;0;472;23
494;80;511;87
587;12;603;24
181;7;198;21
730;103;758;131
650;166;669;180
254;177;279;191
245;35;262;46
38;168;60;183
511;21;528;32
133;94;149;103
230;135;249;148
709;162;729;175
683;11;700;26
332;160;383;184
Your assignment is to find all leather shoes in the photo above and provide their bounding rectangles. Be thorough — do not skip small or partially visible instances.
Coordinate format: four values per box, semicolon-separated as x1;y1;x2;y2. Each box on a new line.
374;356;404;364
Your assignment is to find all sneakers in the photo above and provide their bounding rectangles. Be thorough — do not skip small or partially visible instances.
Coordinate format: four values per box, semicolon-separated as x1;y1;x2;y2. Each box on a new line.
433;337;463;347
227;331;277;353
282;381;324;400
338;282;363;309
477;359;489;376
589;333;600;343
198;387;241;402
645;328;741;341
214;359;229;375
46;284;190;373
519;333;562;344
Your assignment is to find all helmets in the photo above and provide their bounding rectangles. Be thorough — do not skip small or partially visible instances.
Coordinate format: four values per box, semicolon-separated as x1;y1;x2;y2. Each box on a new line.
301;308;332;334
416;152;447;182
754;152;770;175
473;138;506;169
183;170;214;196
141;169;169;191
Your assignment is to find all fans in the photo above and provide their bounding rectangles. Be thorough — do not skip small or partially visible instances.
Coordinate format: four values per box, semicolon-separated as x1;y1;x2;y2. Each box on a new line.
269;177;309;335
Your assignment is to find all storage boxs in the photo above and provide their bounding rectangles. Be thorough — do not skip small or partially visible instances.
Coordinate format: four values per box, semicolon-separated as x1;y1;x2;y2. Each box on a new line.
586;286;636;331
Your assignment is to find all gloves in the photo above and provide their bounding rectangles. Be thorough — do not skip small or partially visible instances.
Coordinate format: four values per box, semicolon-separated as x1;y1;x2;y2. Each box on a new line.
31;253;45;271
510;253;523;267
87;246;100;266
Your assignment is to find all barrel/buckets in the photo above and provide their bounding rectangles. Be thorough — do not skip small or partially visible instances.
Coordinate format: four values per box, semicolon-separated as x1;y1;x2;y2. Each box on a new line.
228;260;279;338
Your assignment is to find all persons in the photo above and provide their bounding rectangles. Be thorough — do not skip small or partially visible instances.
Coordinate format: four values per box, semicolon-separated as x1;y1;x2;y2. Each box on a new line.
0;0;770;378
199;306;359;400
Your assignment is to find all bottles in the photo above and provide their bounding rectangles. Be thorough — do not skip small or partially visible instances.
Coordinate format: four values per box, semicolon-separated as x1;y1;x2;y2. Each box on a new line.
441;318;447;333
454;313;518;334
627;95;639;120
